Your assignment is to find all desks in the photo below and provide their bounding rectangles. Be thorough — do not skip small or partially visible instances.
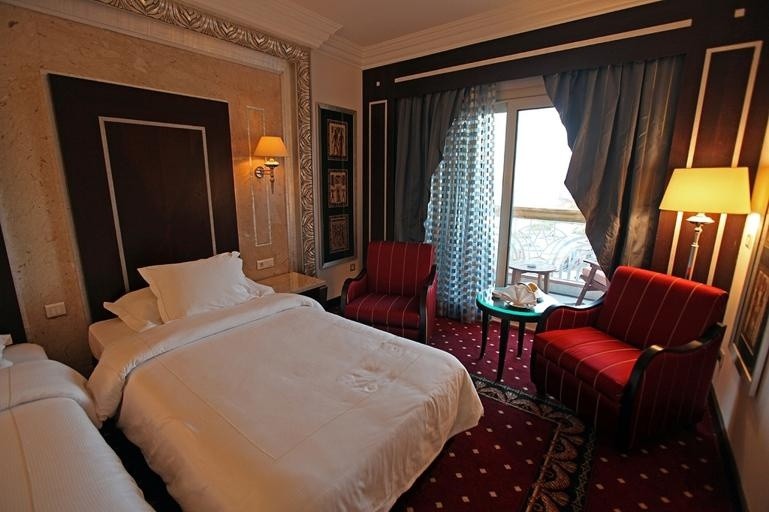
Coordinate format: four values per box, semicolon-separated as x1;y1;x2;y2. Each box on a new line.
476;260;559;382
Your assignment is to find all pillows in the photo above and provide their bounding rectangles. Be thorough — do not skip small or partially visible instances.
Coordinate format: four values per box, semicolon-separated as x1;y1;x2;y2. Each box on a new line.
102;250;263;333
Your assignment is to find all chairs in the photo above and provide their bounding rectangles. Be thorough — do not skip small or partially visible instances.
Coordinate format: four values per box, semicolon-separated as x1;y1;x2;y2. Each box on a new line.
531;264;728;458
340;240;439;346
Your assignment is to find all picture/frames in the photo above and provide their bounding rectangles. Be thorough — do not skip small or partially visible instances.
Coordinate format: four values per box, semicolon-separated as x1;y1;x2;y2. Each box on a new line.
727;195;769;399
314;100;358;272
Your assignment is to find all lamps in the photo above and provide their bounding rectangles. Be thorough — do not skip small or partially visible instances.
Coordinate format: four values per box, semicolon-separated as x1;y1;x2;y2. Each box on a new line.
657;165;751;280
253;135;290;197
